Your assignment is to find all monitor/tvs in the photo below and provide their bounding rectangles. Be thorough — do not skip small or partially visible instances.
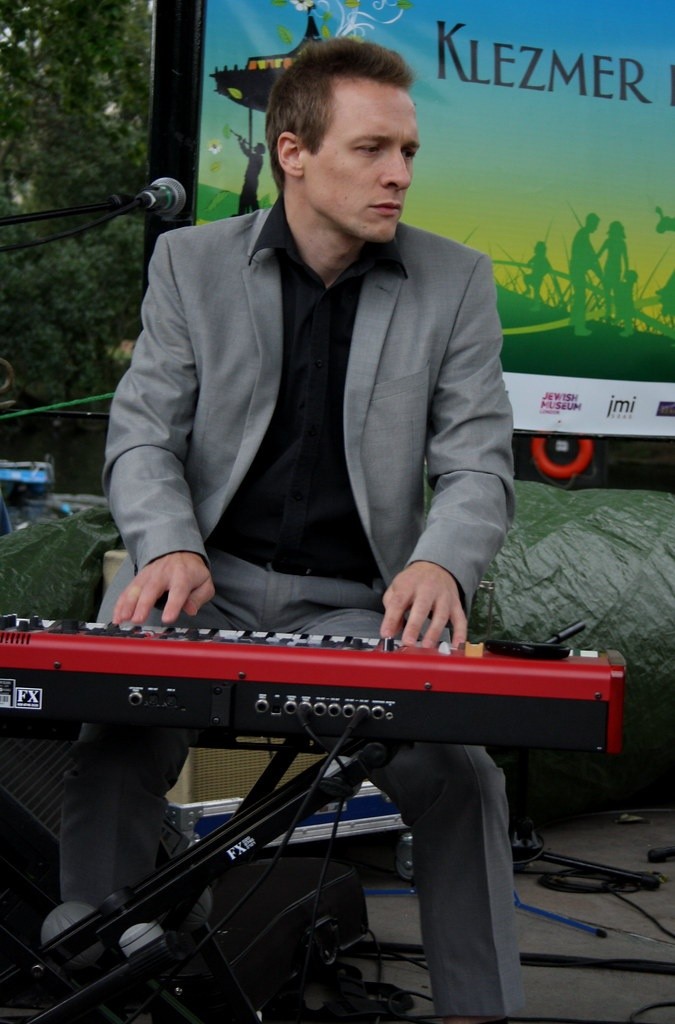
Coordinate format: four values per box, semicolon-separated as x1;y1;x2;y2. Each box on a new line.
146;1;675;495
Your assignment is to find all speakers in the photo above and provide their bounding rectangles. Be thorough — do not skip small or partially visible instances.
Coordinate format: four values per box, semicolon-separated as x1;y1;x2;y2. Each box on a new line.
0;738;79;973
165;736;328;808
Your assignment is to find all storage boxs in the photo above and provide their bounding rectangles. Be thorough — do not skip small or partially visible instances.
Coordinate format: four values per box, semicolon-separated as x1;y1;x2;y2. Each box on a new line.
166;747;408;849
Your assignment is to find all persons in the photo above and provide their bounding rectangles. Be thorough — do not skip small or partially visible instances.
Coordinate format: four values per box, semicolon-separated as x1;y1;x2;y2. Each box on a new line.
36;40;526;1021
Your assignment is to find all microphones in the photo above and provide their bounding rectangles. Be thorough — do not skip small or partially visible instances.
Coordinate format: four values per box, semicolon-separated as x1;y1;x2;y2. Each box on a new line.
135;178;187;217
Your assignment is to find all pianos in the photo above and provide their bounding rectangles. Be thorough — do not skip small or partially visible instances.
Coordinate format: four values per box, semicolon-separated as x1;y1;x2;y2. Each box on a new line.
1;612;628;1024
